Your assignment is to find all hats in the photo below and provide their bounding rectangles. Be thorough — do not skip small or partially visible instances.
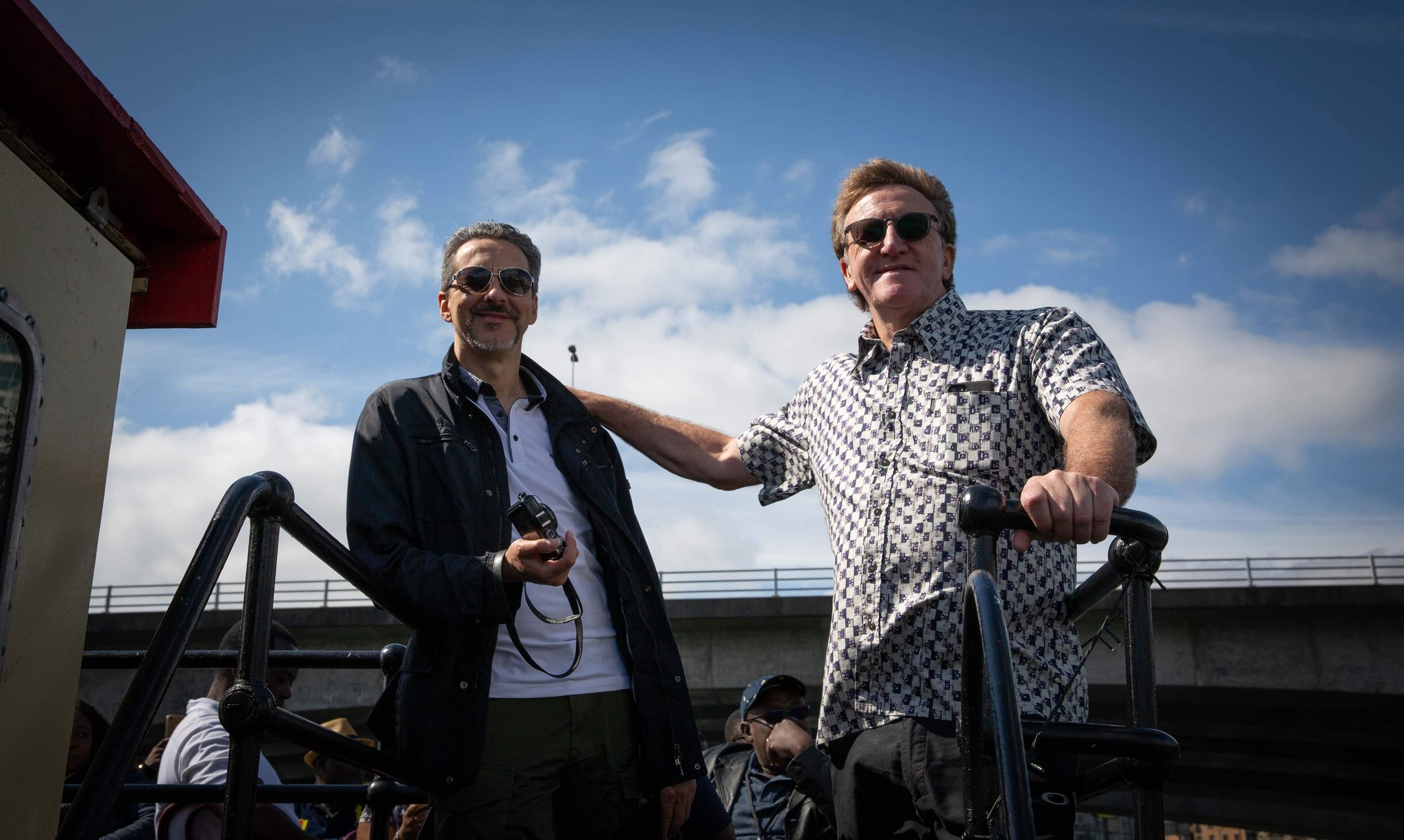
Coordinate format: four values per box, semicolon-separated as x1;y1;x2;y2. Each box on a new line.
305;718;375;769
740;674;807;722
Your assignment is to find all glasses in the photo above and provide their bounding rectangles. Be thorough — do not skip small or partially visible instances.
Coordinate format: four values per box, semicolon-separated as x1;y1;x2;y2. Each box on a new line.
843;212;949;249
745;705;809;724
445;266;536;297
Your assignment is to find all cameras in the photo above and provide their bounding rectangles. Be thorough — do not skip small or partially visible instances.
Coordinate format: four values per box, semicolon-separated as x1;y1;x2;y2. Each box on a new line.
507;494;566;561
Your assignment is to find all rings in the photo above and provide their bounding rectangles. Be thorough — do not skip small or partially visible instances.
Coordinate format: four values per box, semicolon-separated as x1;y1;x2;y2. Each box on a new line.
766;738;769;742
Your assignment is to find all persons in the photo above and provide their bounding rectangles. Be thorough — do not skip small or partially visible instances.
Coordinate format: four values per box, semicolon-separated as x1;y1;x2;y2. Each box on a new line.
561;155;1141;839
694;674;839;840
58;613;444;840
345;219;708;839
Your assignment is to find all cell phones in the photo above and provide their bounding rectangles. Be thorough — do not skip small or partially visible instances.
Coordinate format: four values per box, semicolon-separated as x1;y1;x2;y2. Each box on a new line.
165;715;185;739
